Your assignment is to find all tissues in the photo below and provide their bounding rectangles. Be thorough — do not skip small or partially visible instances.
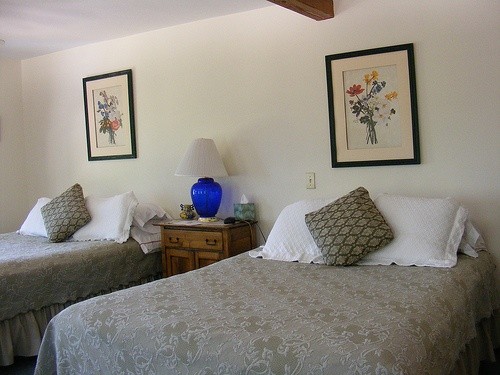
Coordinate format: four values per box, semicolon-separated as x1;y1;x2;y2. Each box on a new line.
233;193;255;221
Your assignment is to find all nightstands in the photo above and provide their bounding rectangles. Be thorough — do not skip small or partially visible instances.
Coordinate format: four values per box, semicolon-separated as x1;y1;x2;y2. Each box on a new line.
153;218;259;278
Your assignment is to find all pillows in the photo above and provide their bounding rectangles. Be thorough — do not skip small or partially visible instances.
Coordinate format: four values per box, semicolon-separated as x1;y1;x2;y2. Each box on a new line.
40;182;91;243
249;193;470;268
16;191;138;244
304;186;394;266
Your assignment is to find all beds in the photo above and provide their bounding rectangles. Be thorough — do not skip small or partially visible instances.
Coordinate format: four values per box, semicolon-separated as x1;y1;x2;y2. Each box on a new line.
0;231;163;367
33;248;500;375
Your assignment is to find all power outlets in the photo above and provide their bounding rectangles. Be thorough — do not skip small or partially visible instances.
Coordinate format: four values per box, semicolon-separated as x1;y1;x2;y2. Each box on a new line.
306;172;315;189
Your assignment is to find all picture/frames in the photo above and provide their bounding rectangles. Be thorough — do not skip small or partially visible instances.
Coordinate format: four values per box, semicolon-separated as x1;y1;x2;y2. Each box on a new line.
325;43;421;168
82;69;137;161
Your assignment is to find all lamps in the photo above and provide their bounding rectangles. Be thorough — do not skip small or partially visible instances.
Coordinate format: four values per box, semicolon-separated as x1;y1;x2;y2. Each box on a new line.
174;137;228;223
